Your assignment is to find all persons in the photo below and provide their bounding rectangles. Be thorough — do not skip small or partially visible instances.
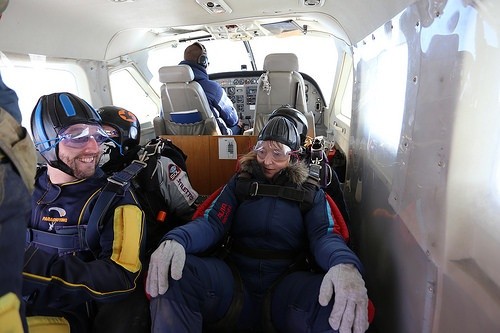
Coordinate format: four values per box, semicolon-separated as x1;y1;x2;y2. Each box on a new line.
159;43;244;135
0;72;39;333
19;92;151;333
145;116;369;333
95;104;200;238
268;104;352;225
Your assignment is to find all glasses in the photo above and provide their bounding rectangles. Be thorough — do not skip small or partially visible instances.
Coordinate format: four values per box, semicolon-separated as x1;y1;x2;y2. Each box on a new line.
257;148;290;162
104;129;120;136
57;124;107;148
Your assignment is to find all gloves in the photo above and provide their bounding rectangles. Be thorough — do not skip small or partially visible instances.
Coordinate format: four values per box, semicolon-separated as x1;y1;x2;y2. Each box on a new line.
146;240;186;297
318;263;370;333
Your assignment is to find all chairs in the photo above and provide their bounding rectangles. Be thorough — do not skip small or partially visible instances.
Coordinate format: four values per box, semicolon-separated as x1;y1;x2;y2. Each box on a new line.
253;53;308;135
159;64;222;137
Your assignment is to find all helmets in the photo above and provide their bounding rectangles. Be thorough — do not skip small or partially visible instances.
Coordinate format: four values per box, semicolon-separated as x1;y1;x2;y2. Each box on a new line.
257;116;300;151
31;91;102;167
96;106;140;156
267;105;309;145
